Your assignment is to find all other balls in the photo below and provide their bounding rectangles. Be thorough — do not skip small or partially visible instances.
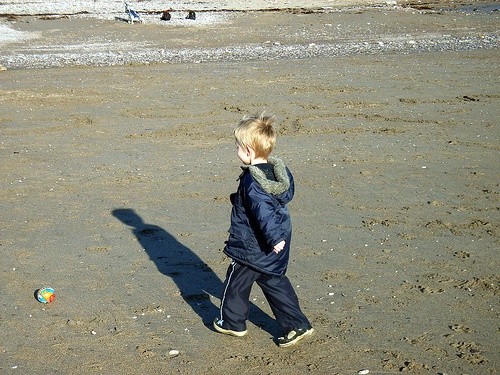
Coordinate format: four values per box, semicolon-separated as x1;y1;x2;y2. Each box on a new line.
36;287;55;302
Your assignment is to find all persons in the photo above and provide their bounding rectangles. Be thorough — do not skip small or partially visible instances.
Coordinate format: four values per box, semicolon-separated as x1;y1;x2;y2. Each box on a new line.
213;109;315;348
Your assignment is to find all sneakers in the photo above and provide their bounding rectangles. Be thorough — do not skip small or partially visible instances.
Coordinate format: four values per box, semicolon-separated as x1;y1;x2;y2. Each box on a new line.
213;318;247;336
278;324;314;347
